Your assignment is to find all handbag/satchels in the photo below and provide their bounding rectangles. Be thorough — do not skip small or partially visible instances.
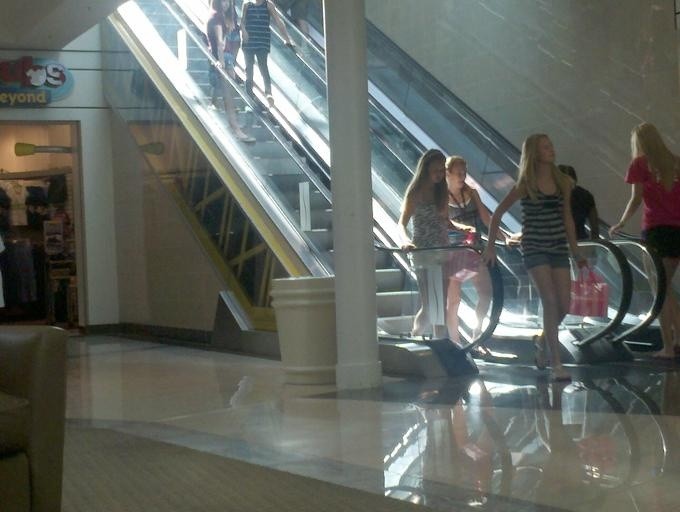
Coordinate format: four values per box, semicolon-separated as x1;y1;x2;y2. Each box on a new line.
565;266;611;318
447;231;483;283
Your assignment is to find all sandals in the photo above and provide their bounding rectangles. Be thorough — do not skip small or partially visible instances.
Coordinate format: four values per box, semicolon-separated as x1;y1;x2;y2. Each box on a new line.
531;334;571;382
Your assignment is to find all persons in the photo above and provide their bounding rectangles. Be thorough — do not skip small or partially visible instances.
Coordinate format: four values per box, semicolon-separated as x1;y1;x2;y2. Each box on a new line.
604;121;679;366
475;132;589;385
404;378;589;509
223;3;242;87
395;148;454;341
237;0;296;108
436;154;523;362
207;0;258;144
508;160;604;250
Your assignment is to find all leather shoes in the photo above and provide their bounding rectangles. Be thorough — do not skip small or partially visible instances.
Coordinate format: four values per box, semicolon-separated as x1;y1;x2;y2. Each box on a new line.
235;134;257;143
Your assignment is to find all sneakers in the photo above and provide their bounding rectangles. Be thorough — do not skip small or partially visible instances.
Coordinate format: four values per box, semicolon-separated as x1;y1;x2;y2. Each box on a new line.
244;95;275;114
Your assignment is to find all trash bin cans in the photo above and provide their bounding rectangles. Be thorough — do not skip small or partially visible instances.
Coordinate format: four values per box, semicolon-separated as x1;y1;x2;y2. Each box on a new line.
268;275;336;384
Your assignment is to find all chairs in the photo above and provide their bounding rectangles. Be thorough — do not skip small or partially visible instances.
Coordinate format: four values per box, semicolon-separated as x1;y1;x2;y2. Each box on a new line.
0;323;69;512
